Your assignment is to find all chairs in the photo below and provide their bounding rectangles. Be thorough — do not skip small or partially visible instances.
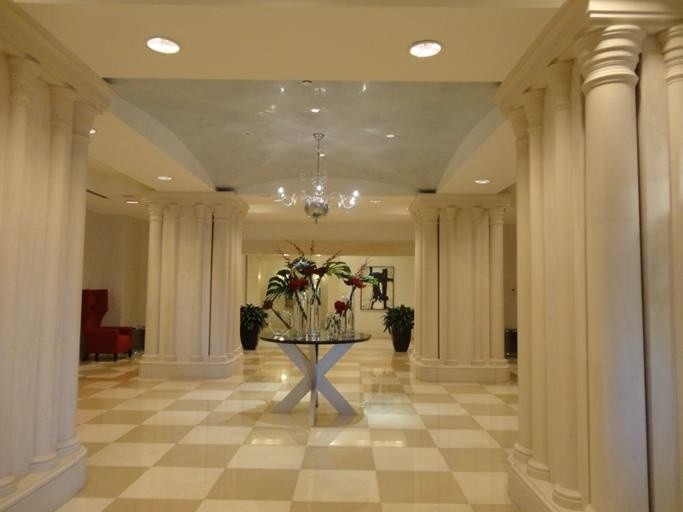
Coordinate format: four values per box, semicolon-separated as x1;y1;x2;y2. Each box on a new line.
79;288;135;362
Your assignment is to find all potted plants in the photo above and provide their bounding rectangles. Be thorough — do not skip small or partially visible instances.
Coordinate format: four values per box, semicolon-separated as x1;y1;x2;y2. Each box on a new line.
239;300;268;350
382;304;414;353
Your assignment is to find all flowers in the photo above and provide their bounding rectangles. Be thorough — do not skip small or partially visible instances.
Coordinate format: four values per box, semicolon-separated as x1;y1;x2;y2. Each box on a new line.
259;237;380;330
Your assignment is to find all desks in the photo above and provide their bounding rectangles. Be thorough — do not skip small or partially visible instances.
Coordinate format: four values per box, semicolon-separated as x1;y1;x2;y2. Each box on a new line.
258;329;370;427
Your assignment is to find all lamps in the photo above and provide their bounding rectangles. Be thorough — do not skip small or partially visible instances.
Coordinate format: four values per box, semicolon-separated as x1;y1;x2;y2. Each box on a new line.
272;132;361;226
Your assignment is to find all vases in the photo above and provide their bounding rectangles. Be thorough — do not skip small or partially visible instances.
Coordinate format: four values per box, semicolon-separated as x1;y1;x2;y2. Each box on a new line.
266;288;354;338
